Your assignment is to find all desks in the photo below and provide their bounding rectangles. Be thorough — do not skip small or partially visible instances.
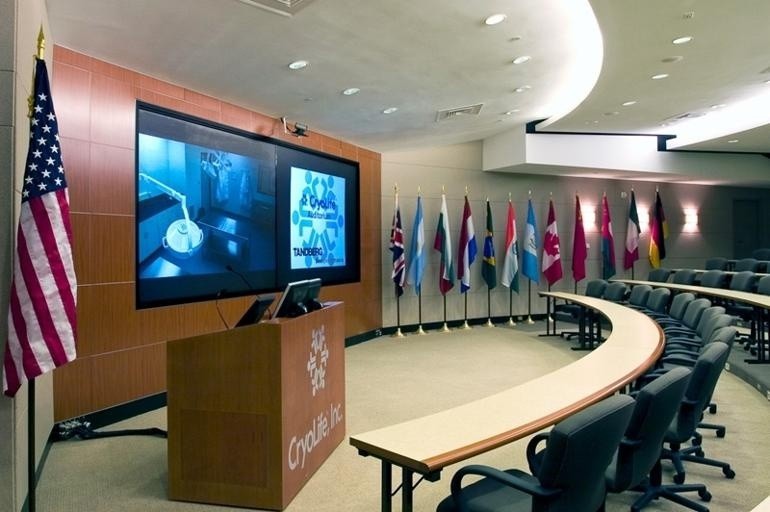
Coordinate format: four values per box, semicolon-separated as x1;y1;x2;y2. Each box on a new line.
607;279;770;364
670;269;770;278
726;259;769;274
349;291;667;511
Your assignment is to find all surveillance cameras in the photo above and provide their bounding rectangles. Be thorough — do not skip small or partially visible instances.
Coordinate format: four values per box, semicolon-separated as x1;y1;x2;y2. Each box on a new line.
294;120;309;137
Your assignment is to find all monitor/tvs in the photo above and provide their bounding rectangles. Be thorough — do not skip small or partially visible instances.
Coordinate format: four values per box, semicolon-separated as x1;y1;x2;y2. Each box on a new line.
136;100;362;310
233;292;277;327
271;277;324;320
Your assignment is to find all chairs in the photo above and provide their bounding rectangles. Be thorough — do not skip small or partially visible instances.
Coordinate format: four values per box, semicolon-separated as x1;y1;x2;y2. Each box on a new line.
560;282;627;344
647;268;670;282
646;292;695;321
718;271;756;316
645;338;729;452
434;394;637;512
664;305;726;332
672;268;697;285
657;298;712;331
618;284;653;307
628;365;712;512
752;248;770;262
734;275;770;356
665;325;741;414
666;313;733;344
632;287;671;313
705;257;728;271
561;278;608;320
700;269;726;307
734;258;758;273
758;275;770;296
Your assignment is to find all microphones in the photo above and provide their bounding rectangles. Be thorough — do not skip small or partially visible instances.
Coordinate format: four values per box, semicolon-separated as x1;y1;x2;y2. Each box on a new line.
216;289;229;330
225;264;272;319
